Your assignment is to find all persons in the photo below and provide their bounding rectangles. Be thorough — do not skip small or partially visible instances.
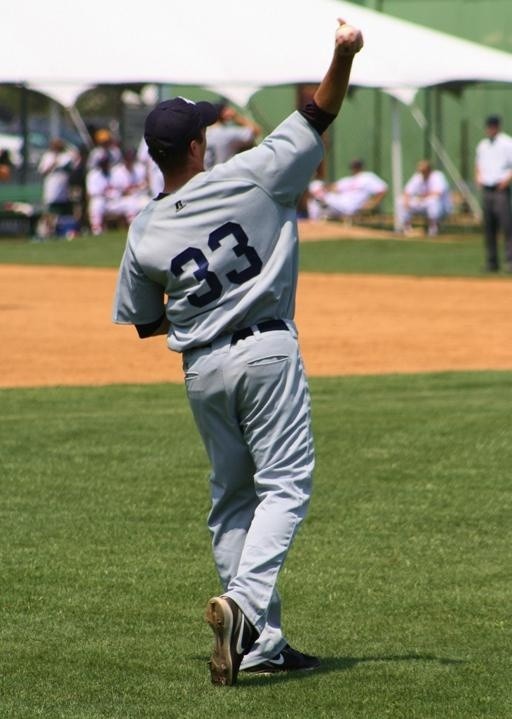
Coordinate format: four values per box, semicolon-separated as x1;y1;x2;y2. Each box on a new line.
113;12;365;685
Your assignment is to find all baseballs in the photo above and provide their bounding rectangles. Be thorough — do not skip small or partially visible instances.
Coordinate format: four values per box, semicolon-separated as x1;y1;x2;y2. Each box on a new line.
336;23;353;41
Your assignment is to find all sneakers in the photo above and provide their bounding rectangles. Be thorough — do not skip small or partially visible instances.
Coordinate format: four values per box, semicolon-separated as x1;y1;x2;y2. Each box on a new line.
243;644;321;672
206;595;257;686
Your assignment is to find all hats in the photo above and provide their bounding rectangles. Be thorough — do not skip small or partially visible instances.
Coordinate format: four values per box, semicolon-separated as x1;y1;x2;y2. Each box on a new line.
144;97;217;163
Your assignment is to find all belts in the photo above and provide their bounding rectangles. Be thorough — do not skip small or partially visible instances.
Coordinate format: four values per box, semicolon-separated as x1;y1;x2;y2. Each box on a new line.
187;319;290;354
